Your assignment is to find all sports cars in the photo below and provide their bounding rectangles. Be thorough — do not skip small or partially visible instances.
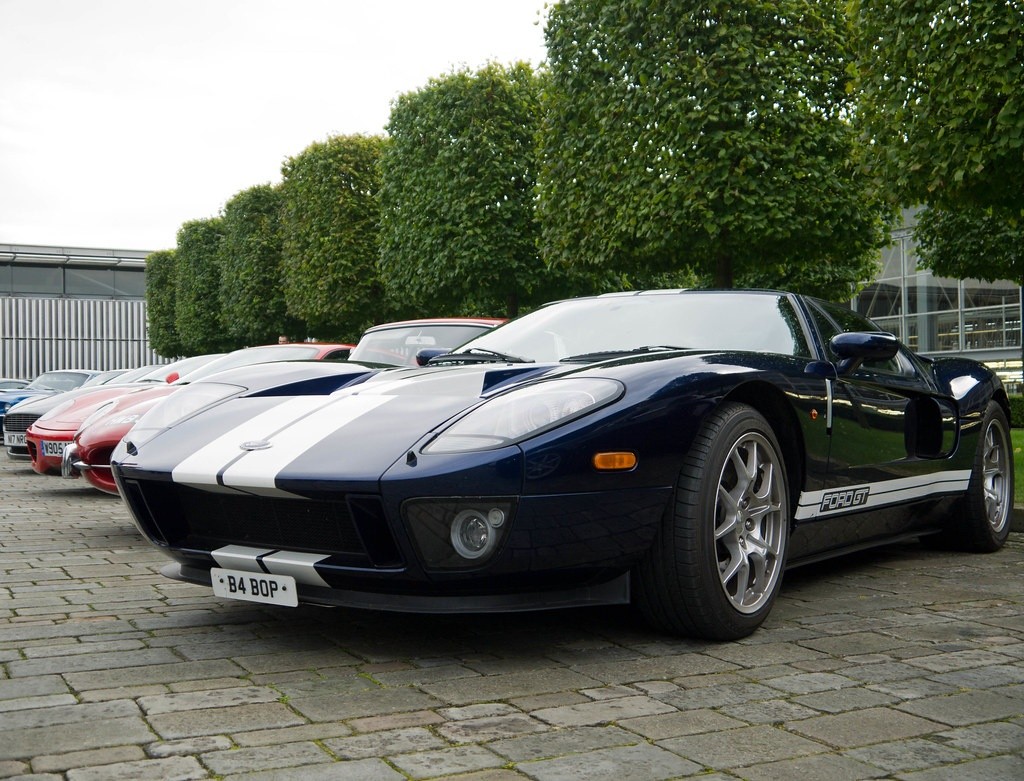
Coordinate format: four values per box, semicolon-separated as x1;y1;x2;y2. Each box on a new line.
106;286;1013;644
60;317;514;497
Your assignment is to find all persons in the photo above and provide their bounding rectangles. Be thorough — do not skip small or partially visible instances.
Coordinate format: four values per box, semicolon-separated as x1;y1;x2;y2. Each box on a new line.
278;336;289;345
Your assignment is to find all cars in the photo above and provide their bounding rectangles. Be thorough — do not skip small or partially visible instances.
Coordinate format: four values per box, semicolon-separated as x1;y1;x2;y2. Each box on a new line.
2;364;168;461
0;378;55;391
0;369;107;445
26;343;419;477
